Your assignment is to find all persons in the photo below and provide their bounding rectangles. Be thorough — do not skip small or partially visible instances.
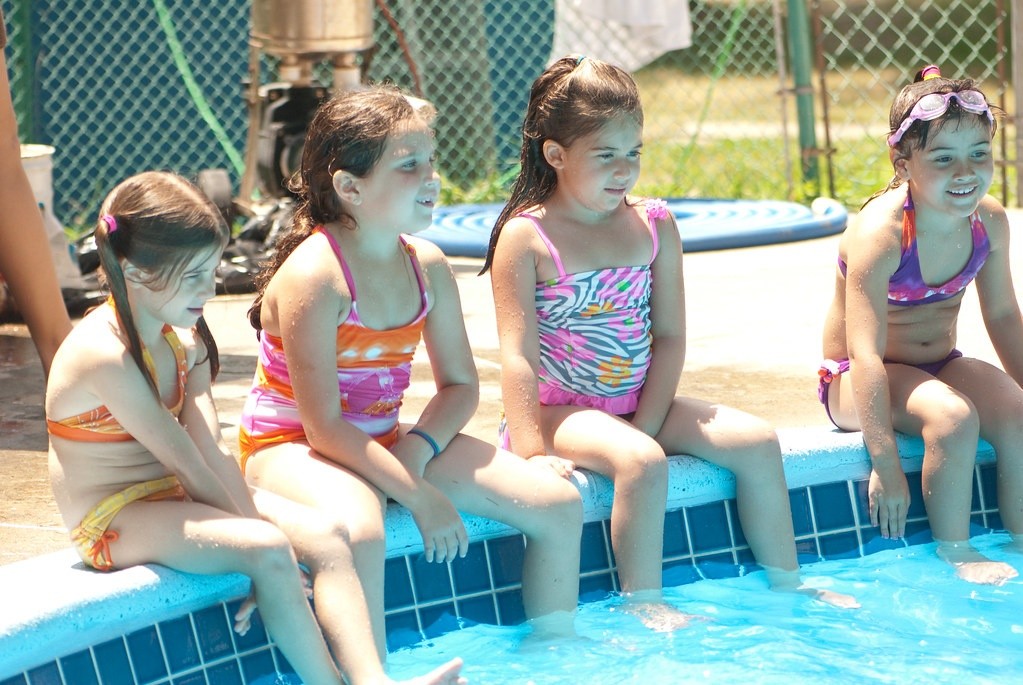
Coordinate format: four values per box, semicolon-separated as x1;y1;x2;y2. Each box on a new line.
44;170;471;685
0;2;74;388
476;56;799;591
817;62;1023;539
237;86;585;655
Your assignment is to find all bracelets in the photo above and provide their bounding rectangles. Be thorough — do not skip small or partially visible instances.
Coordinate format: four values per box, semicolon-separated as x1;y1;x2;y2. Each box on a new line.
407;429;440;458
